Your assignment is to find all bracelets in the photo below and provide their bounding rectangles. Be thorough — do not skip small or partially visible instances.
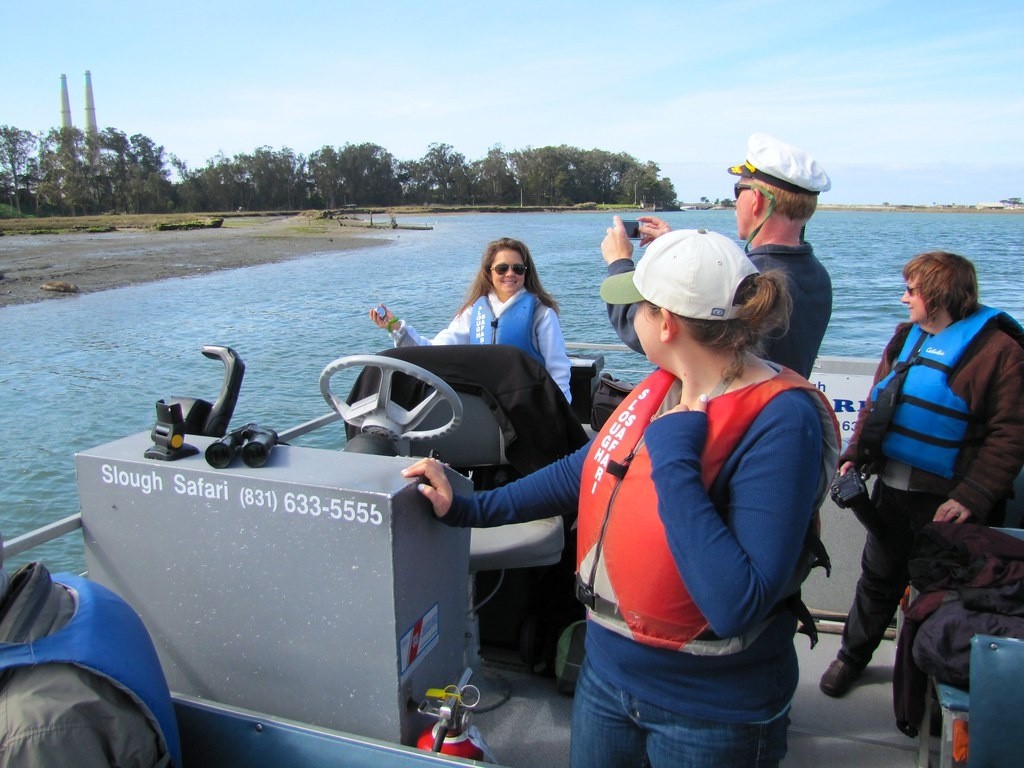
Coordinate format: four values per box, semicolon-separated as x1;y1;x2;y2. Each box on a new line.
387;316;400;334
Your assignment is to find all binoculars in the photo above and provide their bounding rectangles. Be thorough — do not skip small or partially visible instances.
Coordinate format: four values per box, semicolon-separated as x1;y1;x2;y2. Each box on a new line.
204;423;278;469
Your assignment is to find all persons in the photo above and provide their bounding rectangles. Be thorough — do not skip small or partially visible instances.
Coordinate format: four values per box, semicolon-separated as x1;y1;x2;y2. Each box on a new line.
401;226;842;768
595;133;830;381
819;251;1024;701
370;235;575;404
0;558;177;768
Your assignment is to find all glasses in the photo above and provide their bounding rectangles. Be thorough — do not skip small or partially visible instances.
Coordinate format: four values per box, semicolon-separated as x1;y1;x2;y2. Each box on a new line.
733;181;751;199
905;286;918;296
489;263;528;275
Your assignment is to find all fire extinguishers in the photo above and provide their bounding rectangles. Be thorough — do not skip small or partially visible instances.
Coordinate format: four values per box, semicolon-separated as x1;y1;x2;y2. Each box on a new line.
416;666;484;763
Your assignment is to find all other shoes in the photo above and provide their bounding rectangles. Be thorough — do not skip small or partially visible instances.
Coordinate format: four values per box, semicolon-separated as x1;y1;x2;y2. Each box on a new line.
819;658;862;697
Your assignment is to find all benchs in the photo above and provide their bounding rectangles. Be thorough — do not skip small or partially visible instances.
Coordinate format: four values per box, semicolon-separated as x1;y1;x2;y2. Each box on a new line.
169;690;507;768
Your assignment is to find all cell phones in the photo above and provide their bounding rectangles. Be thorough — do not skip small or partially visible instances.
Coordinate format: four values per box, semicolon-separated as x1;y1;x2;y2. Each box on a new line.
613;220;645;240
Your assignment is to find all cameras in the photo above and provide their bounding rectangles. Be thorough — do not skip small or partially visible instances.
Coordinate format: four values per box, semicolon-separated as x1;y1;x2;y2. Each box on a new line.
830;466;872;526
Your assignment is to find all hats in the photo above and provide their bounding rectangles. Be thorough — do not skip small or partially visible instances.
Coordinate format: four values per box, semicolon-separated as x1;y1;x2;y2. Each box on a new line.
599;228;761;320
727;132;832;195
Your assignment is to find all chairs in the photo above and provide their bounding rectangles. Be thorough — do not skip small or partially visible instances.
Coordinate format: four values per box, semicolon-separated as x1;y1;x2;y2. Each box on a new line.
344;344;590;571
968;634;1024;768
916;527;1024;768
